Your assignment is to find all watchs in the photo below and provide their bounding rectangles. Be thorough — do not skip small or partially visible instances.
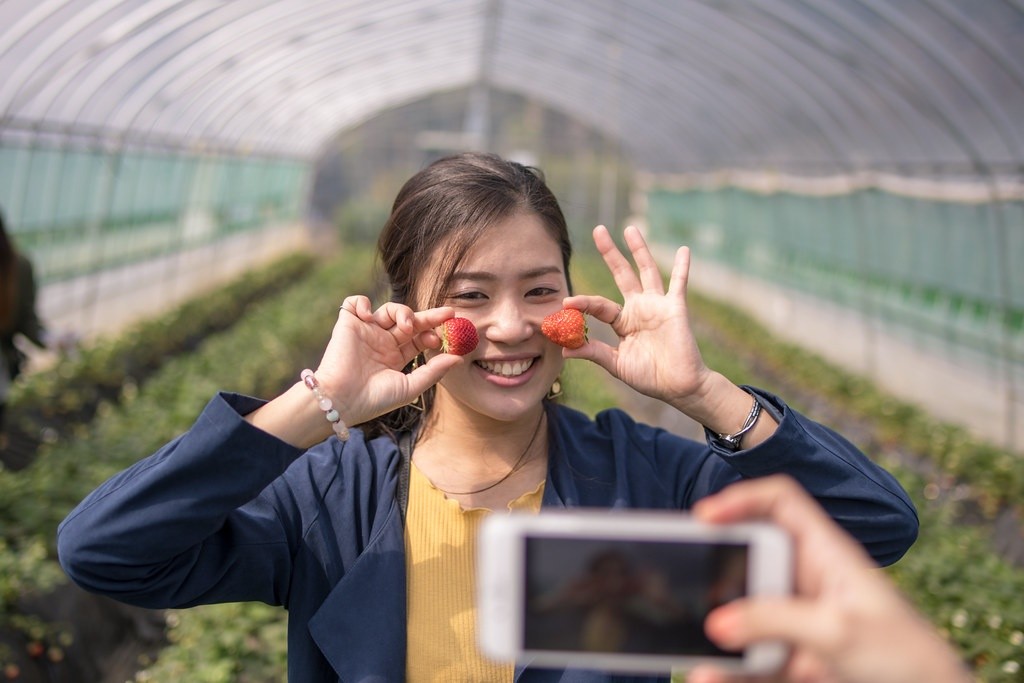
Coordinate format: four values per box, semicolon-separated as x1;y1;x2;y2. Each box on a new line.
715;391;762;451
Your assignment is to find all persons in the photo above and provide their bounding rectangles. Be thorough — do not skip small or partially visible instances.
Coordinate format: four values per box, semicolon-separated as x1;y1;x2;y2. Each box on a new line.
57;148;921;682
686;474;980;683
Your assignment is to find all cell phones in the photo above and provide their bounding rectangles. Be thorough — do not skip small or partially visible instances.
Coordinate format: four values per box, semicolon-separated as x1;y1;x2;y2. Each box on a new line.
479;511;795;675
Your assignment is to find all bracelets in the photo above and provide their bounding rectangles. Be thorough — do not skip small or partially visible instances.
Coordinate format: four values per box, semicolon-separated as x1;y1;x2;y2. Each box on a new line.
300;369;351;443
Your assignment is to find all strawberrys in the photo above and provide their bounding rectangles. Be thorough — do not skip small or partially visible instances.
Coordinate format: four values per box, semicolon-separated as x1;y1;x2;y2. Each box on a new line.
541;307;589;349
440;317;479;356
